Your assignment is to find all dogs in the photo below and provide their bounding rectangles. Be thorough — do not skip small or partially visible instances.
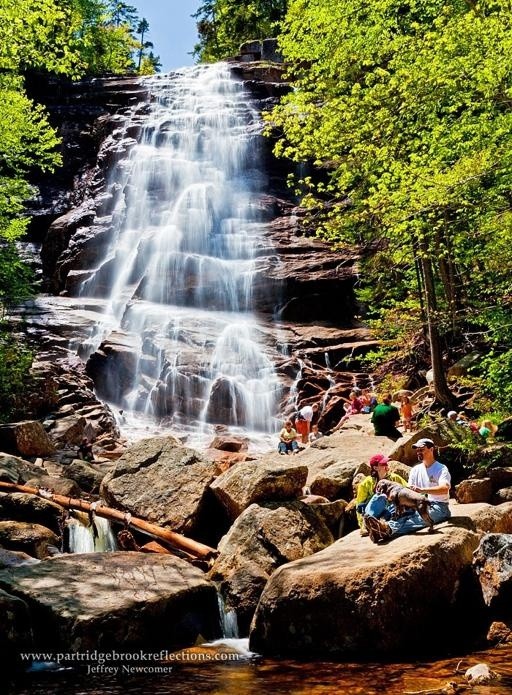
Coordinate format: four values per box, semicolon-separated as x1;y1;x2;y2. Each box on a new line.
374;478;435;534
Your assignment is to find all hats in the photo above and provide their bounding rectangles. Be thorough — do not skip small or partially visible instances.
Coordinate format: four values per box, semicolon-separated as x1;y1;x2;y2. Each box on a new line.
411;438;435;449
370;454;393;465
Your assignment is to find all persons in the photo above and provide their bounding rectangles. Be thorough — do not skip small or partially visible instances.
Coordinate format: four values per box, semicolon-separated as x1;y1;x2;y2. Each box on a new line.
356;453;407;536
277;387;498;455
365;438;451;545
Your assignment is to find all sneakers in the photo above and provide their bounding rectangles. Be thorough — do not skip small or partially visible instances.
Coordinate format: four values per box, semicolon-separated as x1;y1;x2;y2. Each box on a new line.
360;516;393;543
